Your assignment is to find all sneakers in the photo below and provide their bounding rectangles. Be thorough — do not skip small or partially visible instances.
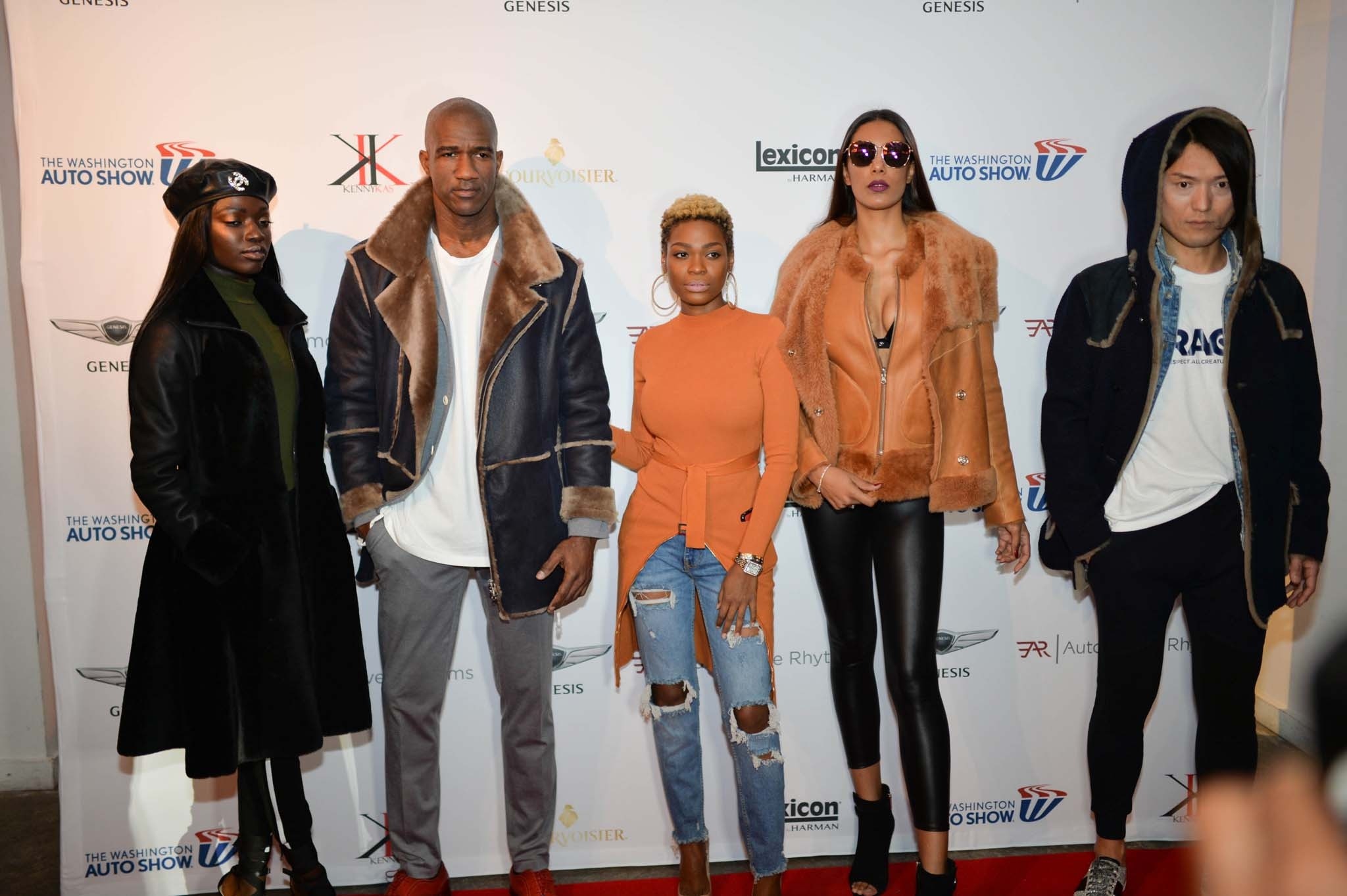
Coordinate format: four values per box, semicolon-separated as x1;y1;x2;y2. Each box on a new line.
1073;855;1127;896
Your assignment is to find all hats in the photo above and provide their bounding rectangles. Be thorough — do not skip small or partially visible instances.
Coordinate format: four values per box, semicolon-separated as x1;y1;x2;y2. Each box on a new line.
162;157;278;226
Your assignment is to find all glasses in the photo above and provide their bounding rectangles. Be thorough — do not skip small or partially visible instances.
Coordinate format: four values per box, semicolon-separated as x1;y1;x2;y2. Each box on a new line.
843;140;914;169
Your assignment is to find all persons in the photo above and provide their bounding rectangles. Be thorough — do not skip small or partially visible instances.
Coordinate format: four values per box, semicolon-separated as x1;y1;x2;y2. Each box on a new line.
771;109;1032;896
1037;106;1332;896
325;98;619;896
606;193;799;896
114;156;371;895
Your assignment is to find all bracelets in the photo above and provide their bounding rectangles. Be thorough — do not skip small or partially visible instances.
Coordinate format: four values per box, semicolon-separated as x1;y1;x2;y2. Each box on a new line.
736;552;763;564
817;464;832;493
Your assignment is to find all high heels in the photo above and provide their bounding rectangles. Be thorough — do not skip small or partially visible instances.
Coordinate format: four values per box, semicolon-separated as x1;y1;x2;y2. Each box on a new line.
751;873;783;896
282;863;336;896
914;857;957;896
848;784;895;896
218;848;270;896
677;836;713;896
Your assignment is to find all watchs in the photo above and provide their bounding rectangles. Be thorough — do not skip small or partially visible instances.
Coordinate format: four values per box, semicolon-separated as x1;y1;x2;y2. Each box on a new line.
734;558;763;577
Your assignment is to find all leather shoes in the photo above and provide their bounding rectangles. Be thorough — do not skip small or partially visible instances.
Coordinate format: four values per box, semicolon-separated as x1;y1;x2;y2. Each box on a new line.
509;865;558;896
385;861;451;896
